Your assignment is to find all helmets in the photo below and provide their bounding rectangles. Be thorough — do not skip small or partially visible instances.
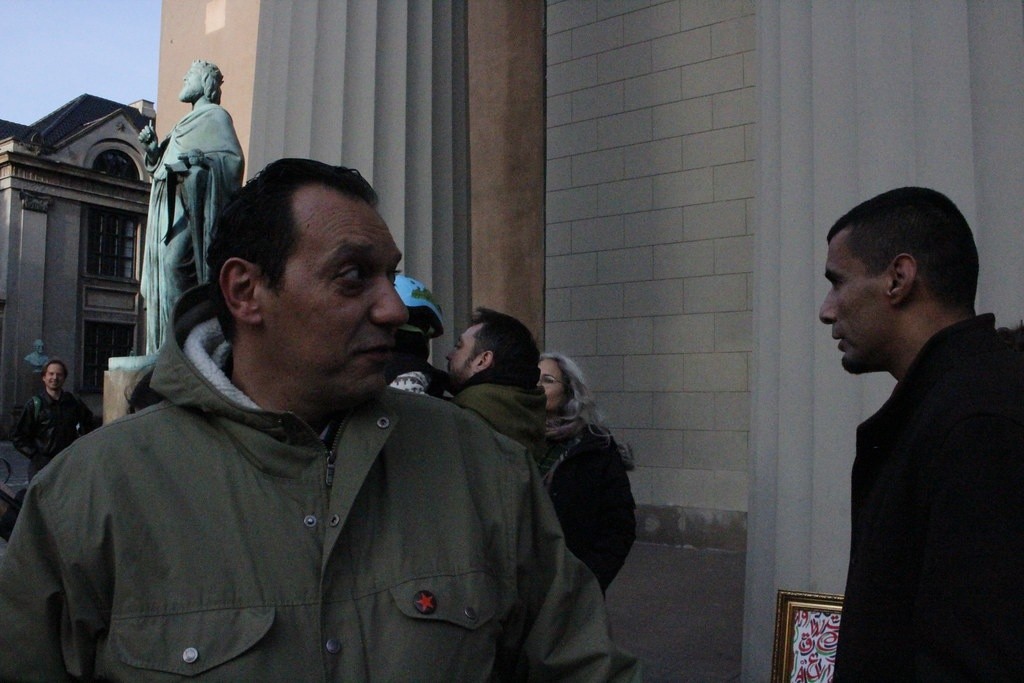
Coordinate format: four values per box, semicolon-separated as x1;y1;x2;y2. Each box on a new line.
394;274;444;337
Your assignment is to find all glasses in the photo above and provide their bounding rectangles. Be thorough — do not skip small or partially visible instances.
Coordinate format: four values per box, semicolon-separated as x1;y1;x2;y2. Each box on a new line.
539;374;569;385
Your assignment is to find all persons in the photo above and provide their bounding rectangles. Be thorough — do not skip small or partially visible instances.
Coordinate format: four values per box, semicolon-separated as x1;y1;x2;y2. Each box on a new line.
1;158;642;682
816;184;1023;683
389;275;639;602
24;339;50;366
137;56;249;361
11;359;95;483
